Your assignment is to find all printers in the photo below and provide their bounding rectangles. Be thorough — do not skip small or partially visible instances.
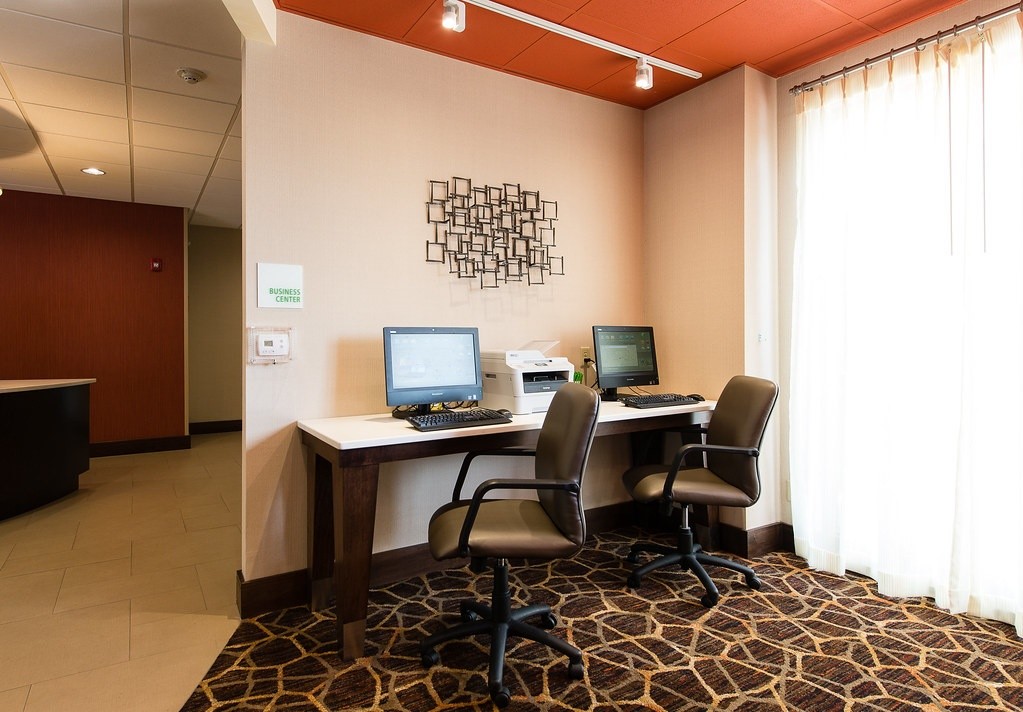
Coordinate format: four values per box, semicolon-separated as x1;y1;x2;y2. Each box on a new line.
479;349;574;414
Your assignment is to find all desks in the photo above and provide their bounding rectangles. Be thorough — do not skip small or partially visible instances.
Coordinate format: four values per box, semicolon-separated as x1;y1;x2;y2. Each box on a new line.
295;397;718;662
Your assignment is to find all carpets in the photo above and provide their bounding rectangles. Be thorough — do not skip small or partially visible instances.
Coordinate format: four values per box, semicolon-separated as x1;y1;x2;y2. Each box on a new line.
171;517;1022;711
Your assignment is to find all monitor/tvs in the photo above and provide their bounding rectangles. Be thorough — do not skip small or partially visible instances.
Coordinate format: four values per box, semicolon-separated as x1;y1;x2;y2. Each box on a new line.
383;327;484;419
592;326;659;401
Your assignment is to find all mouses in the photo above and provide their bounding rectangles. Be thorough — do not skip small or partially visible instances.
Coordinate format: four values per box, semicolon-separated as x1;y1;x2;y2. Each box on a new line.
687;394;705;401
497;408;513;418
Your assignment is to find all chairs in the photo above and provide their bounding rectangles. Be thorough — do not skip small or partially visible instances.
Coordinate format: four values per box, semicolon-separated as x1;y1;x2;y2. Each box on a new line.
620;374;780;607
421;382;602;709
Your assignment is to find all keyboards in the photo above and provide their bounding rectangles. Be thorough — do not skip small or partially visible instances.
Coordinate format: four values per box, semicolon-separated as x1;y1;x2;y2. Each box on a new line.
623;393;698;410
407;408;513;431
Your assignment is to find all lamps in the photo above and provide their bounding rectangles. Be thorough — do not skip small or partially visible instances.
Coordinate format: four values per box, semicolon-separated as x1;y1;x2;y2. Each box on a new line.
441;0;466;33
634;56;655;91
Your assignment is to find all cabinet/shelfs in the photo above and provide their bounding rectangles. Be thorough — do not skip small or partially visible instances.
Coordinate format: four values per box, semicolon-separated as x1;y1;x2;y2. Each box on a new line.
0;377;97;525
